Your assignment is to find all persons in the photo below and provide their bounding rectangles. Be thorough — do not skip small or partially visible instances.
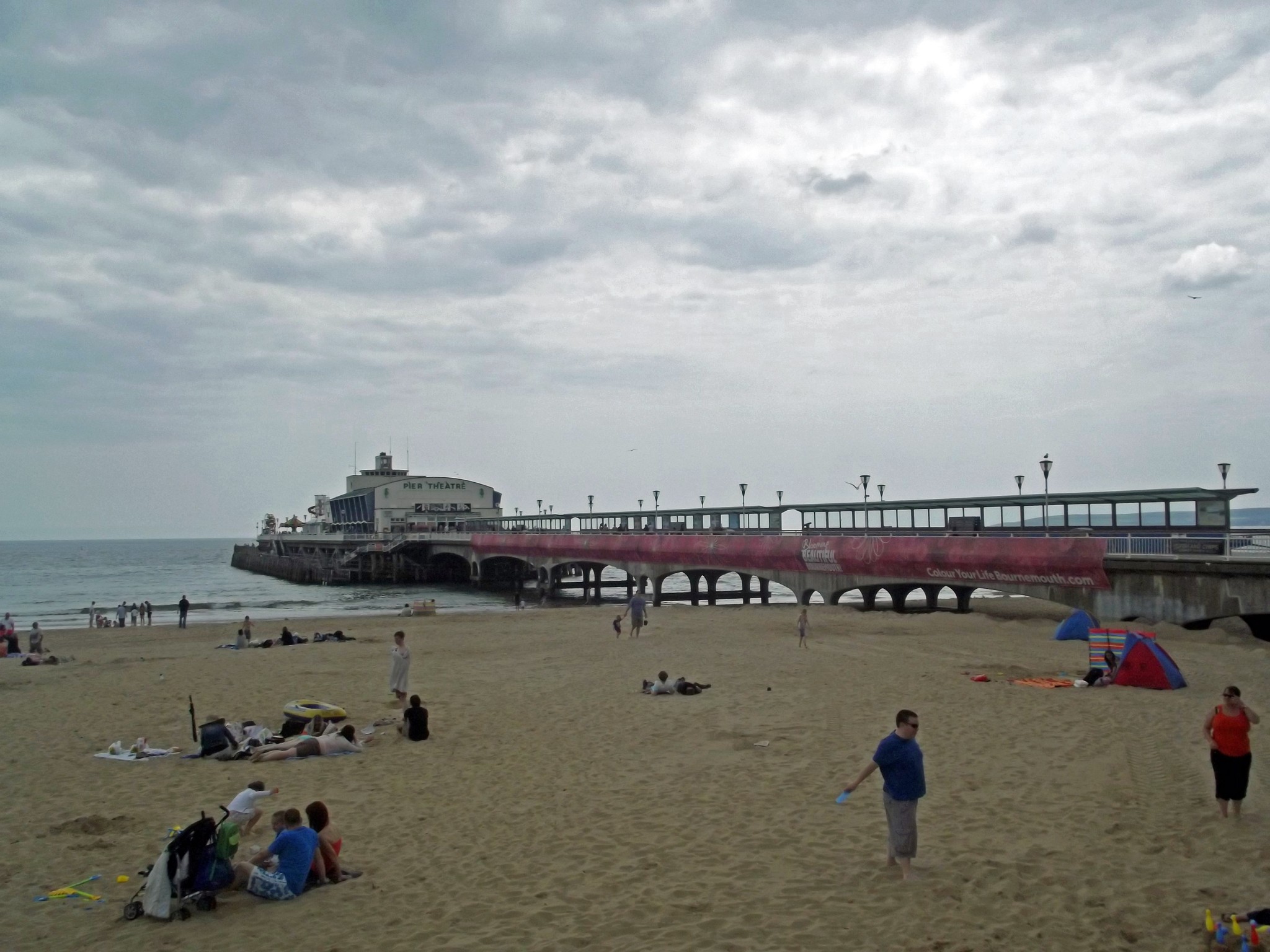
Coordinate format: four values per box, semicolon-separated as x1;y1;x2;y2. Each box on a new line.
513;589;521;611
1202;685;1260;822
208;780;364;902
842;709;926;884
950;521;958;537
396;694;430;741
0;612;76;667
612;614;626;639
640;670;675;696
89;600;153;629
398;604;412;617
231;615;356;650
796;608;811;649
389;631;410;722
519;598;526;611
262;523;726;540
672;676;712;696
1220;907;1270;926
624;590;648;640
196;714;375;764
1104;650;1121;679
177;595;189;629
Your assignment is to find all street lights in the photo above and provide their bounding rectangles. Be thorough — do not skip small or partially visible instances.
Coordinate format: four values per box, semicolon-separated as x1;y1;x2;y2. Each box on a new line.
495;503;501;533
543;509;547;514
638;499;644;511
1217;463;1231;488
860;475;871;535
1014;475;1025;495
877;485;885;500
652;490;661;531
699;496;705;508
1039;460;1053;536
514;507;518;528
549;505;553;514
519;511;522;516
256;510;347;535
776;491;784;505
433;504;469;535
588;495;594;534
537;500;543;533
739;484;748;535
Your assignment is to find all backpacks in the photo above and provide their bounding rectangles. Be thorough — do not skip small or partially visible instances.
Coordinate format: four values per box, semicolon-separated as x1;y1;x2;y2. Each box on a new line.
215;818;239;860
191;843;236;891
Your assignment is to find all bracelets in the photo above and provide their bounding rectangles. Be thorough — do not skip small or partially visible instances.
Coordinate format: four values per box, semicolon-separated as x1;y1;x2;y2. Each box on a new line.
1241;705;1247;711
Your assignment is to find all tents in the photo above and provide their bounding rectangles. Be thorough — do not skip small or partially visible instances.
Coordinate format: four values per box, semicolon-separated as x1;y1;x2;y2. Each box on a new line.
1053;609;1101;641
1086;626;1188;691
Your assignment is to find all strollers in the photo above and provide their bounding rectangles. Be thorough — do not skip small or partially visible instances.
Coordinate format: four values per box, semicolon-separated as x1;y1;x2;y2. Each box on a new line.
123;804;232;923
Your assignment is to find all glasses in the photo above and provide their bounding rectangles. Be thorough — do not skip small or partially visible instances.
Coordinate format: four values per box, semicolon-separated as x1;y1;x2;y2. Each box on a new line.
1223;693;1234;698
905;723;919;728
